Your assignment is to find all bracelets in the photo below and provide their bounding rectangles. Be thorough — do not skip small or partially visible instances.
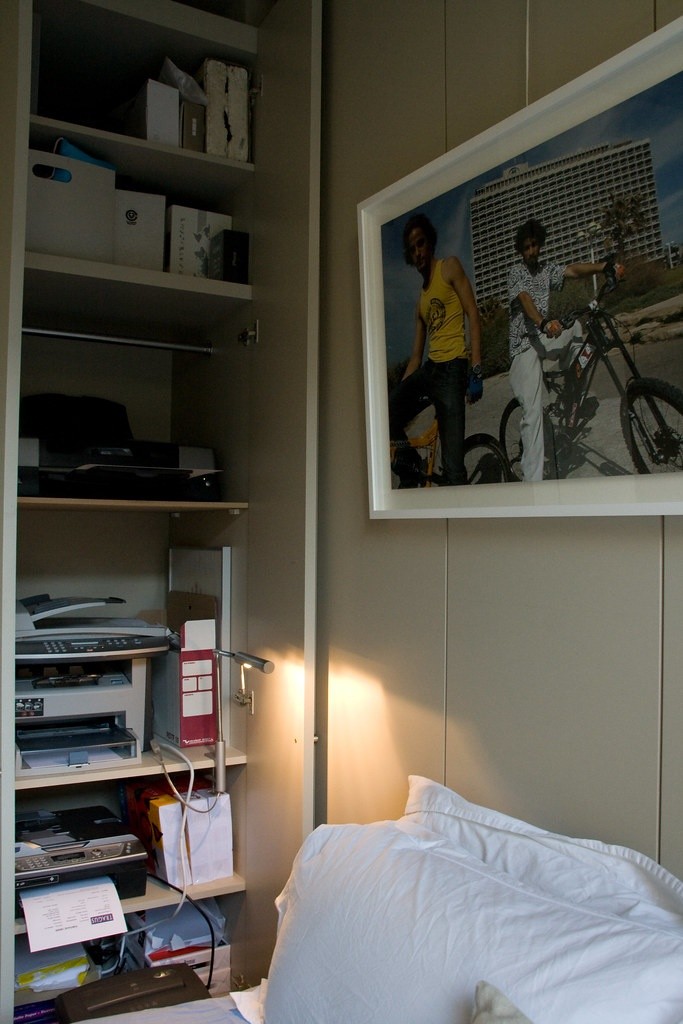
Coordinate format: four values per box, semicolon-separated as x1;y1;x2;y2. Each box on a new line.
539;319;548;331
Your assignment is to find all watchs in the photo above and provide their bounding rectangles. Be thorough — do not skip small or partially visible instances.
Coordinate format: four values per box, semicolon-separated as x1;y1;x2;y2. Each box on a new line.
470;364;482;375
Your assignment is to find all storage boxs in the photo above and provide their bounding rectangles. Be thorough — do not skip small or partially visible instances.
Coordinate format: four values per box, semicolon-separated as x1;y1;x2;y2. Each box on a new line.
24;78;250;284
122;908;232;999
136;590;219;749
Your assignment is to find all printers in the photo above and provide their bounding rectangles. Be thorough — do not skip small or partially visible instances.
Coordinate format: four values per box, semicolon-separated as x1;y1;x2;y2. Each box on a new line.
16;806;149;917
53;963;212;1024
15;593;170;778
18;394;224;502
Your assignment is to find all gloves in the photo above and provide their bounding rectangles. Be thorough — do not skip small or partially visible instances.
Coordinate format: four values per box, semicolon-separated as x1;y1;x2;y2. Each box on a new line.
465;371;483;405
602;262;626;281
540;319;563;339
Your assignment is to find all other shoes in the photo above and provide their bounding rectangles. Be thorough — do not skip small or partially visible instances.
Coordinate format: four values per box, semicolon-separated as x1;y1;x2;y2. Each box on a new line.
564;396;599;427
399;448;422;488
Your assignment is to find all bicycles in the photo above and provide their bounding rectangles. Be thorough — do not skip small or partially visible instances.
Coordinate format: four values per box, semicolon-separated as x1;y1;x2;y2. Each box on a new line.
500;276;683;482
389;395;513;488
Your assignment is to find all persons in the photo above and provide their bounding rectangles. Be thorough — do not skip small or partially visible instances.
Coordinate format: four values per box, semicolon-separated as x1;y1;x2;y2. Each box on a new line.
388;214;483;489
502;217;624;482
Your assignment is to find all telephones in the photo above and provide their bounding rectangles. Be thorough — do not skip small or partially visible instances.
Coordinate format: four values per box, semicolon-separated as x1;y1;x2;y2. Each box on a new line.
58;961;213;1022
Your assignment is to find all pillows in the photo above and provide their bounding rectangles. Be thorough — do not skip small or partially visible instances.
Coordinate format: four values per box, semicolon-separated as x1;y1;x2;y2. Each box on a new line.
262;775;682;1023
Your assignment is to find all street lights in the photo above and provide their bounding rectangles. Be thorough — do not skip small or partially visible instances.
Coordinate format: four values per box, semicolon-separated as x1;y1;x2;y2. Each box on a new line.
665;241;675;270
576;222;602;297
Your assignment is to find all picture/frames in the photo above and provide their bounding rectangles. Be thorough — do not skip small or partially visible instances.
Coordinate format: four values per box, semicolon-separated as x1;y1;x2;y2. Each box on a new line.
355;12;683;521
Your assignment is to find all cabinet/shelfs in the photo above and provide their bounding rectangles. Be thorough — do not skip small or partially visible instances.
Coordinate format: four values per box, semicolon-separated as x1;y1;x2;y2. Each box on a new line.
1;0;321;1023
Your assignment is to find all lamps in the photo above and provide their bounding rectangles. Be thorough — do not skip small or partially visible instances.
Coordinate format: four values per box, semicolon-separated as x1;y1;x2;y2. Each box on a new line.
211;648;276;794
